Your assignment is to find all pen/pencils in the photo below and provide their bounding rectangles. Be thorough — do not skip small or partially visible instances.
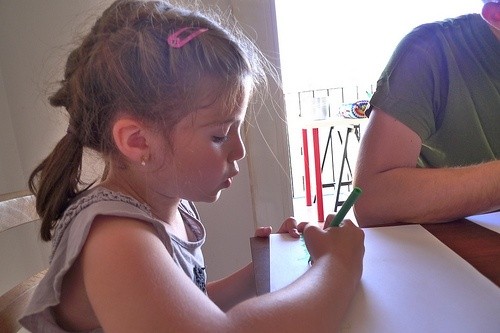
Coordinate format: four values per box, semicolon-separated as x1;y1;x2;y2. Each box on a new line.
308;188;361;265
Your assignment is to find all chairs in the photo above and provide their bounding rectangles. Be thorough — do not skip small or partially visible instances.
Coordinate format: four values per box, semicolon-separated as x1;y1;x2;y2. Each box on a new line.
1;186;53;333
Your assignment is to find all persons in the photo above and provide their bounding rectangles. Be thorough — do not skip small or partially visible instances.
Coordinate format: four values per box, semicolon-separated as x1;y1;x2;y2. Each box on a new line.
18;0;366;333
352;0;500;228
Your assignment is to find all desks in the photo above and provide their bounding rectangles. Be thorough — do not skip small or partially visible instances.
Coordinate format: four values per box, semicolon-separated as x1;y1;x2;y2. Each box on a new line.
247;218;500;332
303;117;370;214
